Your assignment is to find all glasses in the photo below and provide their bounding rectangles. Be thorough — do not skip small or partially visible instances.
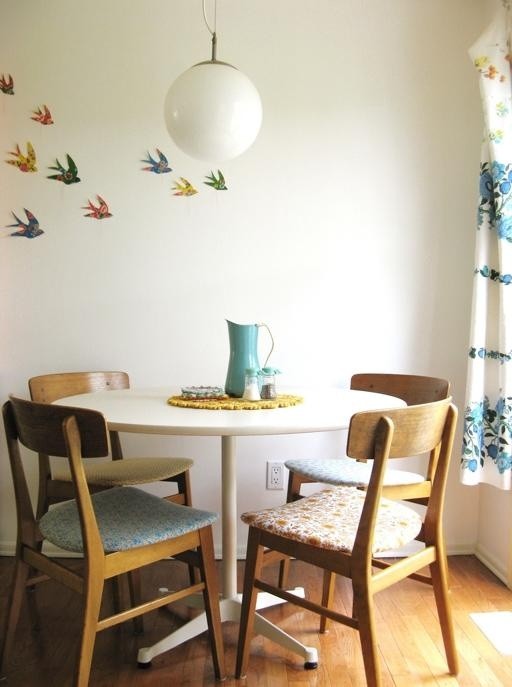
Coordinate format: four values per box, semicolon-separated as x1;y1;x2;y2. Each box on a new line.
161;0;265;162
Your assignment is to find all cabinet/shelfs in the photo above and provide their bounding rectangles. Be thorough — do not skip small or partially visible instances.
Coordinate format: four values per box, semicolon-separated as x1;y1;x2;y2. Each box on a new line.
257;367;277;400
240;367;262;401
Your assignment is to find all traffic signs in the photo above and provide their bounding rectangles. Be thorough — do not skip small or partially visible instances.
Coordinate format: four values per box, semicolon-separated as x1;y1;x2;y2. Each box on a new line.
265;461;284;490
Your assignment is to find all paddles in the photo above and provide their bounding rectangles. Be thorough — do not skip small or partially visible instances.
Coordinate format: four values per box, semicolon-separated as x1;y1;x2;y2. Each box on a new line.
469;610;512;657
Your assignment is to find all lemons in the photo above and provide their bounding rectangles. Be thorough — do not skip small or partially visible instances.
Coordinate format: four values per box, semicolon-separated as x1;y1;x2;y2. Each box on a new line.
222;315;276;398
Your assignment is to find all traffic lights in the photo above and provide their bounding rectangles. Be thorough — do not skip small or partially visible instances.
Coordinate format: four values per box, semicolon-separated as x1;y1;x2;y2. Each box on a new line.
241;486;423;555
52;455;194;485
284;453;425;488
38;487;220;552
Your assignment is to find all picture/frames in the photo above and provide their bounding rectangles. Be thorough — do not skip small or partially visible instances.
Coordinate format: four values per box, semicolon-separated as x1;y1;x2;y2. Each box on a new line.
50;384;405;670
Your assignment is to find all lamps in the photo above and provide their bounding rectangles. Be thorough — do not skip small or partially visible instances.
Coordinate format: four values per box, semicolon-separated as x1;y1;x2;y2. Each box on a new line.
24;370;204;624
280;374;448;634
0;394;223;687
236;396;459;686
